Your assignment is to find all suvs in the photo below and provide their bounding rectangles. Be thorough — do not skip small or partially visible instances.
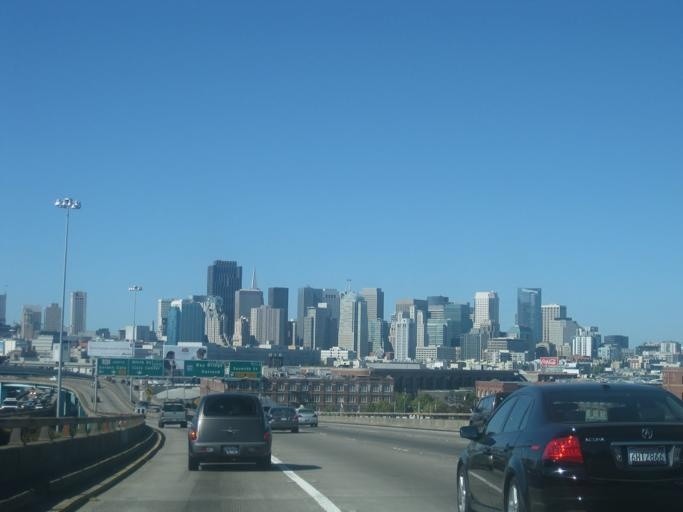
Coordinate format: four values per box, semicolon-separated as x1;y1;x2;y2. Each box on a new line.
159;401;187;428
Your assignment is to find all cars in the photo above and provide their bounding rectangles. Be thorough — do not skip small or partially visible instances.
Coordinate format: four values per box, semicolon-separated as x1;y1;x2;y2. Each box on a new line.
0;388;43;412
262;403;319;432
455;381;683;511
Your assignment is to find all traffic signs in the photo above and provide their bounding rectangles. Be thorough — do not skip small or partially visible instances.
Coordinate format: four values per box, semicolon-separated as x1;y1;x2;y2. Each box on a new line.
128;359;164;378
96;358;129;377
184;360;224;378
229;361;263;380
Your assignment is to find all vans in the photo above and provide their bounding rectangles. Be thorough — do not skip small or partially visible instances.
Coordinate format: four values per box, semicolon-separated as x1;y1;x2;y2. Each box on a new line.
187;392;272;472
469;391;511;433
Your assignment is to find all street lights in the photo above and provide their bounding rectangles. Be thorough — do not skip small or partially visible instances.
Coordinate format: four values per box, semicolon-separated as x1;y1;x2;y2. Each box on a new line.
54;195;83;434
128;285;143;402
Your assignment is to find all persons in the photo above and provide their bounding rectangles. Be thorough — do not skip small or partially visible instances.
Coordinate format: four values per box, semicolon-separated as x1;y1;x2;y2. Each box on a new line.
195;347;206;359
163;351;176;370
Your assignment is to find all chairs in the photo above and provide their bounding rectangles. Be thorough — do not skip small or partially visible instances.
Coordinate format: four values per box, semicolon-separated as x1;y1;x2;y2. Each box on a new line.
554;402;584;422
605;406;636;422
637;406;665;421
